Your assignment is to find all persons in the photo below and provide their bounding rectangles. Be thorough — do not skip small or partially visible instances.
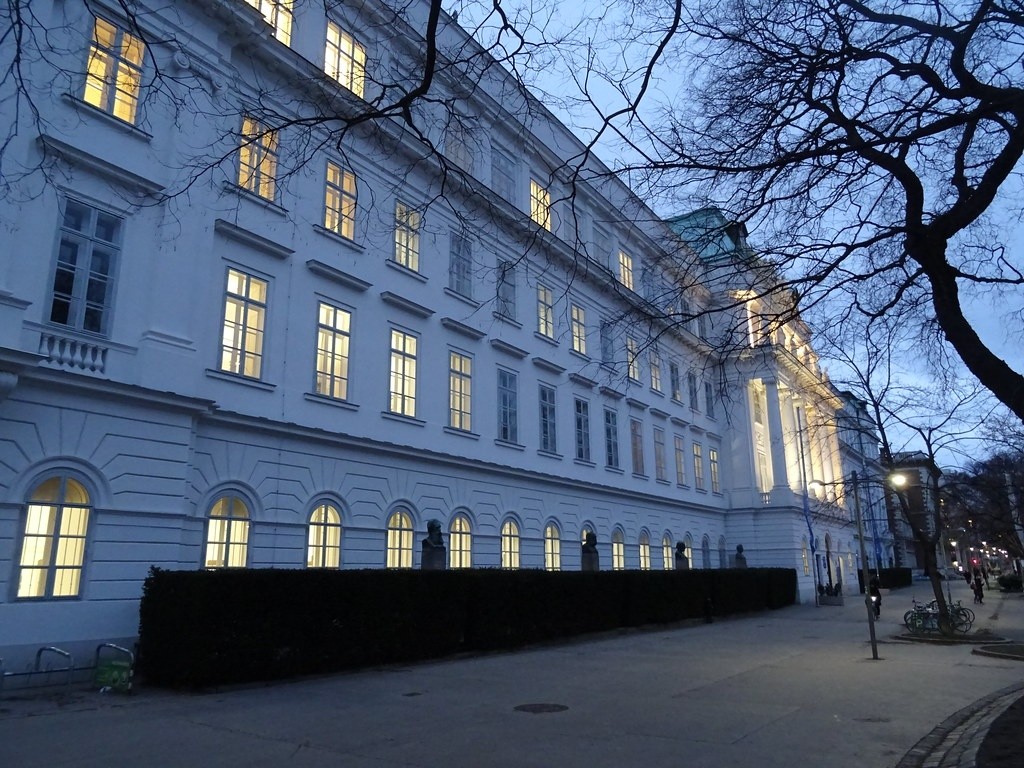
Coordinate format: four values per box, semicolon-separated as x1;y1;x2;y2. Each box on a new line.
963;568;971;584
980;566;990;590
974;573;984;604
869;585;882;616
973;564;982;578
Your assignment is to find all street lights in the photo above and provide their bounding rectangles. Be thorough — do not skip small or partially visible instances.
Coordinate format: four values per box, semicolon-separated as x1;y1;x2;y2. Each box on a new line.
850;470;910;659
939;537;1009;605
